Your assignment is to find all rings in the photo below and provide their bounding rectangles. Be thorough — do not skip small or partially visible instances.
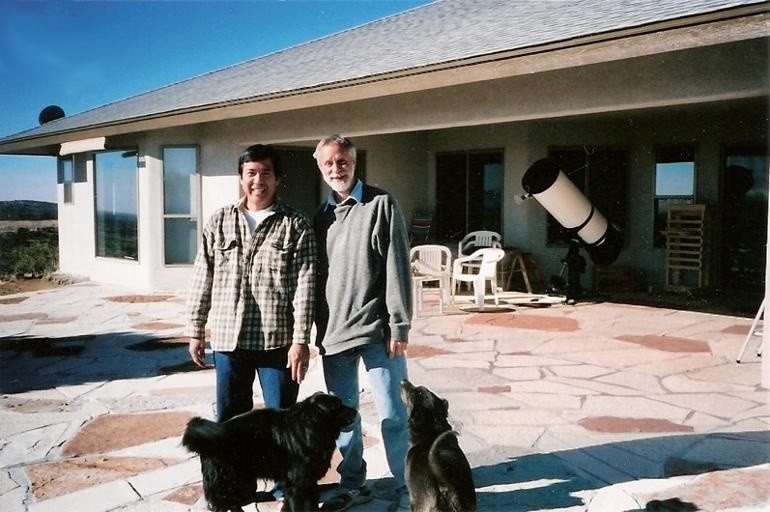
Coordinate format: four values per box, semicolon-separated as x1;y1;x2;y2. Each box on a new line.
302;365;307;371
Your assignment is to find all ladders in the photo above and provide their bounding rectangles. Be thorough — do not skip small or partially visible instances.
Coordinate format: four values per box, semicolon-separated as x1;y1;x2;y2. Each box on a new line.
505;253;545;295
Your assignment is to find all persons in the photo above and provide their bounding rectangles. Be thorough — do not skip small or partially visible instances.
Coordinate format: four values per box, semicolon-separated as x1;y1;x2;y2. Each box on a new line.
184;144;320;512
309;134;414;512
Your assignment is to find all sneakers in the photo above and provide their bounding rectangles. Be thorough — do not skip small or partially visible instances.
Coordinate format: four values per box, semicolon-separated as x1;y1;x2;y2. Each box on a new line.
316;478;373;512
396;485;412;512
277;496;287;512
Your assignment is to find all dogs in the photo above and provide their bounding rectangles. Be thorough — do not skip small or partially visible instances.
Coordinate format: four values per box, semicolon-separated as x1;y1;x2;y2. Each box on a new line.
178;390;358;512
399;378;477;512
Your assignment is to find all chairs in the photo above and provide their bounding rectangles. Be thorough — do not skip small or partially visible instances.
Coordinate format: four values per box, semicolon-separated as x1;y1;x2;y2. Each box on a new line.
408;245;452;316
457;229;503;292
407;207;434;240
452;248;510;312
